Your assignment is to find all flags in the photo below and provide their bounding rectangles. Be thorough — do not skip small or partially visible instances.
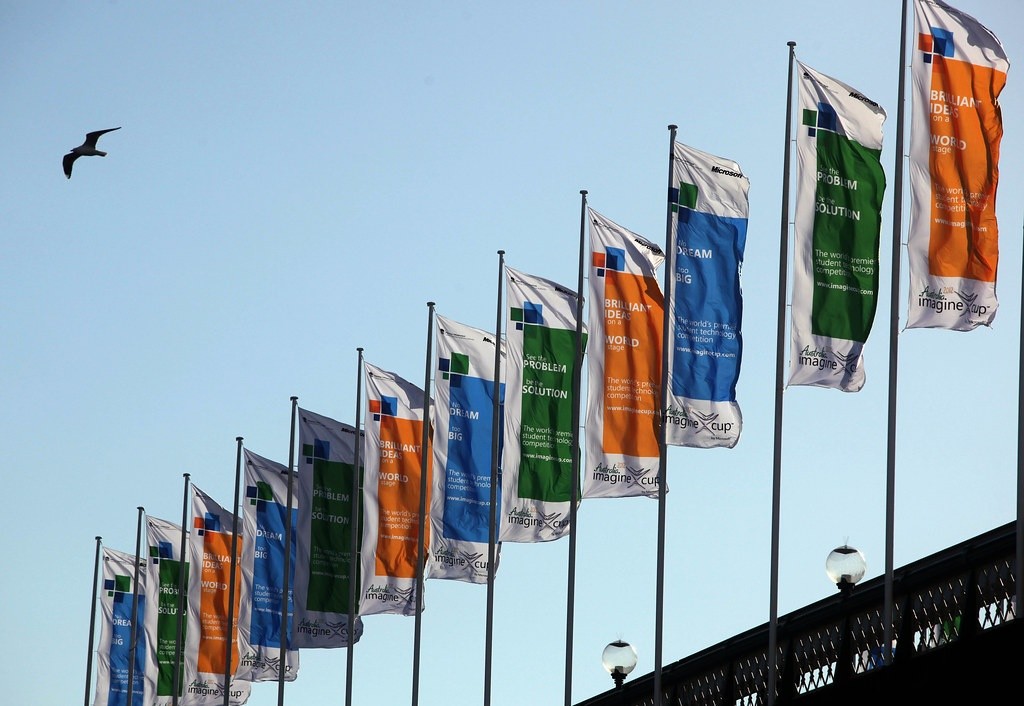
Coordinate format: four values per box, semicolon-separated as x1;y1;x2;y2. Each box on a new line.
500;265;588;544
94;546;146;706
142;516;190;706
667;143;750;449
288;406;364;649
233;447;300;683
177;482;251;706
581;205;669;498
788;62;887;394
907;0;1010;333
425;313;502;584
359;362;434;616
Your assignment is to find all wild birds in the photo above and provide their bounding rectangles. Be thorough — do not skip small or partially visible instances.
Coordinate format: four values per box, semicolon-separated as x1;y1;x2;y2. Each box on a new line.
62;127;121;179
